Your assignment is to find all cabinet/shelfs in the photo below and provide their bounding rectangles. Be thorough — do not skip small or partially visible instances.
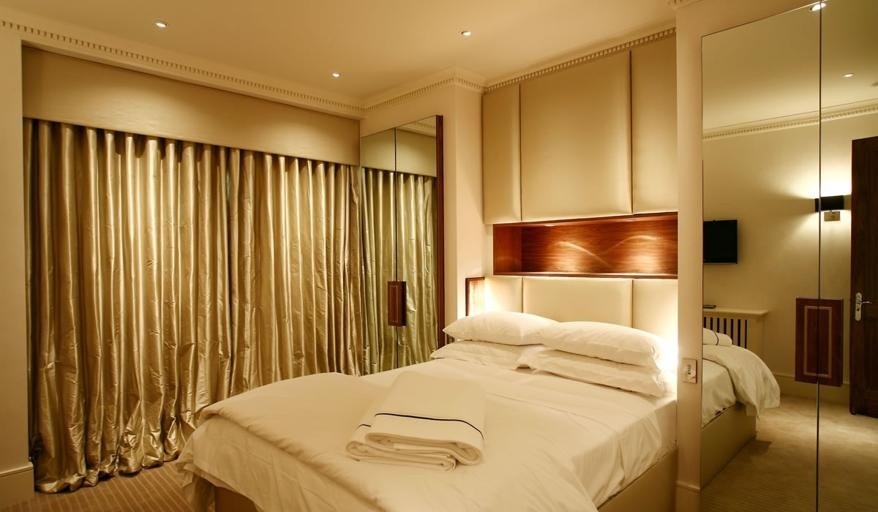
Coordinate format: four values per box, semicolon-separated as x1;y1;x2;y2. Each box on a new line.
358;115;444;376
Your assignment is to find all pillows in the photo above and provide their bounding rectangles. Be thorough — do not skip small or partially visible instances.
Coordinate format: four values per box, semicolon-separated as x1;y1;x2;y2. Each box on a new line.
431;309;671;400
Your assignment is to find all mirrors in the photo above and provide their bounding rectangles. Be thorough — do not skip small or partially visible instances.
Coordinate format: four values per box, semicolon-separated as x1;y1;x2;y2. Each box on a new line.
694;7;876;510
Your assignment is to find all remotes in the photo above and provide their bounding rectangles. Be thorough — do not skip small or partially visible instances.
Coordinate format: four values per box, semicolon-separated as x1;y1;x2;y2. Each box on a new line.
704;305;715;308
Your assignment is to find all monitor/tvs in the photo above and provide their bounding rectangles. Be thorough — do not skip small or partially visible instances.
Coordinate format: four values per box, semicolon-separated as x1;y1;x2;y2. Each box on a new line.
703;220;738;265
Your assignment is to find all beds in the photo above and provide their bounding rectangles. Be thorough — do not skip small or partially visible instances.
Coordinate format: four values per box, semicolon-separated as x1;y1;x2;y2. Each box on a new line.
700;325;782;492
174;279;682;511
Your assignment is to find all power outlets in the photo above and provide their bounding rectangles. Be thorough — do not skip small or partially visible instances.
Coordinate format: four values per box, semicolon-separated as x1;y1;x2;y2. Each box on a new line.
680;358;698;383
